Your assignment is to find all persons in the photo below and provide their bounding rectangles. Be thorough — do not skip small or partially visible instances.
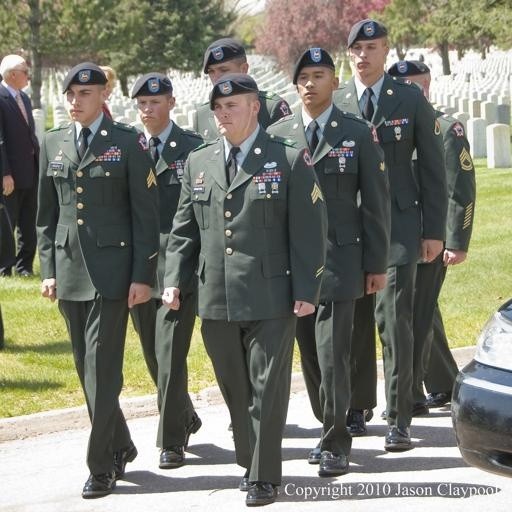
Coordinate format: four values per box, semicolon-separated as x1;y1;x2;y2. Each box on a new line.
331;19;446;451
125;73;203;469
98;66;115;120
191;38;292;144
382;59;476;420
160;72;328;506
0;54;40;276
38;63;159;497
266;47;392;478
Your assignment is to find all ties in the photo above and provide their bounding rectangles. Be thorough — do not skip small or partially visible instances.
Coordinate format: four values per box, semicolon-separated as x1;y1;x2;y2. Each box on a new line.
361;88;374;123
225;146;240;189
305;119;319;154
75;127;91;162
148;137;162;163
16;90;29;125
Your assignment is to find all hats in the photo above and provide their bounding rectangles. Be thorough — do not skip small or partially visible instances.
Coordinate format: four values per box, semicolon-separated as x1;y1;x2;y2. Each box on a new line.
62;62;107;95
291;48;335;85
209;72;259;110
131;72;174;99
347;19;388;49
202;37;246;74
388;60;431;77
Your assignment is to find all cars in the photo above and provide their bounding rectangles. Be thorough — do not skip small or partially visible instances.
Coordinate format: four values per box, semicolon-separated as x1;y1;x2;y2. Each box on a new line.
450;299;511;484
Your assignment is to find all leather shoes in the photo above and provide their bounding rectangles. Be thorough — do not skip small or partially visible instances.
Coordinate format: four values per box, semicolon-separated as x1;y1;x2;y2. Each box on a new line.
346;408;374;427
307;443;321;463
245;482;278;508
384;422;411;451
112;439;138;481
426;391;453;407
381;399;430;419
185;412;202;451
82;467;116;498
239;468;255;491
15;269;37;280
345;404;366;437
319;451;349;476
159;443;185;468
